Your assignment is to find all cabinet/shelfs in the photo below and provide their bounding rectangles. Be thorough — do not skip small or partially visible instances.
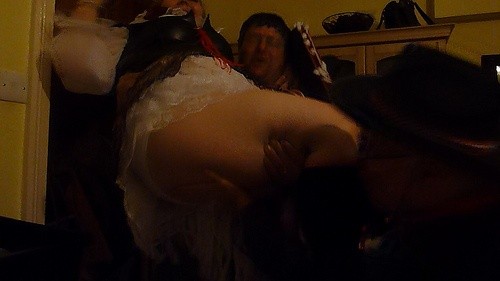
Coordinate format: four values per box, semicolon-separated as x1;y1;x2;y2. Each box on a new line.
226;21;456;86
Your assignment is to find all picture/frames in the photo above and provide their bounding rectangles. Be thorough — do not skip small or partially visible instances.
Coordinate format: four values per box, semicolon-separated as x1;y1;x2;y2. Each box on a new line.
425;0;500;26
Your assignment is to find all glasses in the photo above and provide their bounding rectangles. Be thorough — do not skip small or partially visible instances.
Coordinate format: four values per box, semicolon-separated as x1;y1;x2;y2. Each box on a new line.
354;132;417;163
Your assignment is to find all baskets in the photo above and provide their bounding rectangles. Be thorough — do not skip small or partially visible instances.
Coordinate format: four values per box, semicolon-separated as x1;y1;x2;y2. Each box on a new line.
322;11;374;35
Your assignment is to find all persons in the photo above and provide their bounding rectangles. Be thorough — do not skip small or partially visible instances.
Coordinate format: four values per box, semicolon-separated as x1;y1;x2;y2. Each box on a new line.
264;45;500;281
51;0;364;281
237;13;307;98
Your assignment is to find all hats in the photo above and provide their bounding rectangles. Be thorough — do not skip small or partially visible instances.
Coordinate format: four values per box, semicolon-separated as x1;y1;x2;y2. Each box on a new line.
329;43;500;182
285;21;327;91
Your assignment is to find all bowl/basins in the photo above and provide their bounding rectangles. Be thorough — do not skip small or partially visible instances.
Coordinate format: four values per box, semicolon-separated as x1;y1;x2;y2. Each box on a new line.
322;12;375;34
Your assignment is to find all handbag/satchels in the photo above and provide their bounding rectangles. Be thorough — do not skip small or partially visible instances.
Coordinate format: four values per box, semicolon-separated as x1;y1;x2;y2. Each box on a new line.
376;1;434;29
